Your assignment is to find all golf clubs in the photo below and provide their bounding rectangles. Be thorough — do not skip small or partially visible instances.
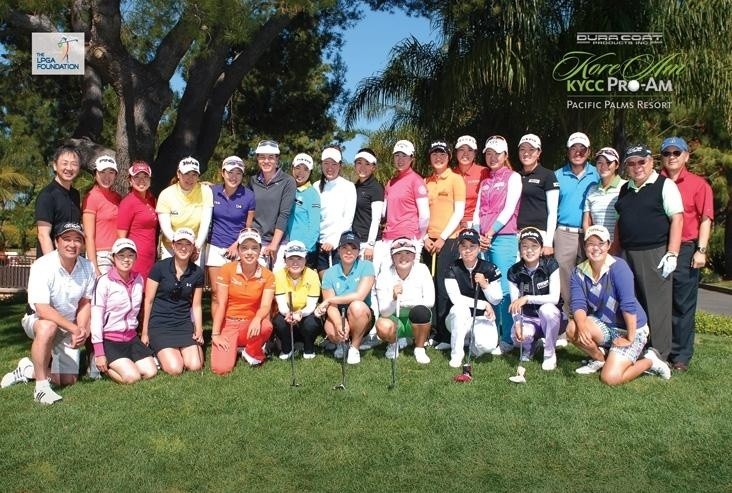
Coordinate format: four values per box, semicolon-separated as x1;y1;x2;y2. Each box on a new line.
509;281;525;384
335;307;345;389
288;291;300;387
455;282;480;382
388;279;402;389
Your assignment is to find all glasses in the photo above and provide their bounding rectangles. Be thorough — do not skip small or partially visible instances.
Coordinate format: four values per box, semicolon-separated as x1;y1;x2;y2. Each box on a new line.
239;227;260;234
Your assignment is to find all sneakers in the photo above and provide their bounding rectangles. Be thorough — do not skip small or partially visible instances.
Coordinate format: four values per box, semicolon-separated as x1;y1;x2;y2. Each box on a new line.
33;385;63;405
243;334;673;382
0;356;33;389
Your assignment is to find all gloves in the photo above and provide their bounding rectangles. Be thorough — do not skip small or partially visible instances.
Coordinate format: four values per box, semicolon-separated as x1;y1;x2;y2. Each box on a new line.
656;251;680;279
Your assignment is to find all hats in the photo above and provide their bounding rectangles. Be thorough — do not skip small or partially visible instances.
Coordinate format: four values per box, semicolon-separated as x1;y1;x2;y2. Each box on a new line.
584;225;611;243
389;238;417;255
339;232;361;250
518;228;544;248
284;239;307;258
54;221;86;240
236;228;263;245
94;132;692;180
110;237;137;255
173;226;195;245
455;228;481;246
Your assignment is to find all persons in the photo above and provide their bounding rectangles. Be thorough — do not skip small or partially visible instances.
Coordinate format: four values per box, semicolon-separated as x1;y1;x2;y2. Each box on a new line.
568;224;673;386
24;130;717;378
90;237;159;383
1;220;97;403
